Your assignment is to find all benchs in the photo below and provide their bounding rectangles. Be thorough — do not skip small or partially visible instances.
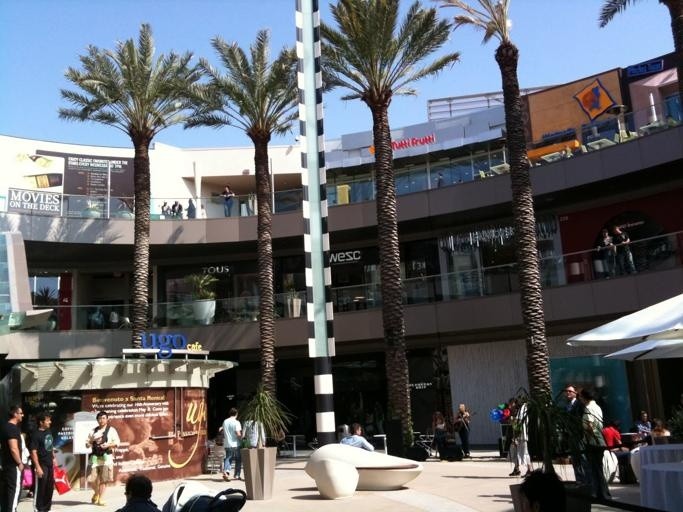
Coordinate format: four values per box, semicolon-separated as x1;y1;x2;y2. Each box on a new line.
305;443;422;492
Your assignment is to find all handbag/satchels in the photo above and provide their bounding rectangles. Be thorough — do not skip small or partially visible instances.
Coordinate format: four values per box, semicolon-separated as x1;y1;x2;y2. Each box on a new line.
22;465;34;488
53;470;72;495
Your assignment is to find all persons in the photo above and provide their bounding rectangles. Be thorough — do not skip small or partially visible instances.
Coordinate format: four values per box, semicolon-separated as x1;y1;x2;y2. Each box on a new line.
612;225;636;275
431;403;472;458
219;185;235;216
438;172;445;188
93;308;120;329
597;229;616;280
504;383;672;481
1;406;162;512
212;408;286;481
339;423;375;452
562;145;581;159
162;199;206;219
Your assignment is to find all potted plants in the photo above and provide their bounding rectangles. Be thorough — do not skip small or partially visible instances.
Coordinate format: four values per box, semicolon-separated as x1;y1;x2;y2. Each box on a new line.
34;286;58;331
241;379;294;501
188;274;217;326
286;288;302;320
508;387;615;512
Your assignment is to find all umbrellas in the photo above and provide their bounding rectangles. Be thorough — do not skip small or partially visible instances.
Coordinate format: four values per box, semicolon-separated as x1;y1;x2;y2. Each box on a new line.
562;290;683;346
603;340;683;360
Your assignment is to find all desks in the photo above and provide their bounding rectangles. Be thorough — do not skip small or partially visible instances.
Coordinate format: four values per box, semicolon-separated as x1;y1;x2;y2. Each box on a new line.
639;444;683;464
640;462;683;512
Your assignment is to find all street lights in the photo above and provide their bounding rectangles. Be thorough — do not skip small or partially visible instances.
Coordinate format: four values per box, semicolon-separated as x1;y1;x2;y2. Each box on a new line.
105;158;115;219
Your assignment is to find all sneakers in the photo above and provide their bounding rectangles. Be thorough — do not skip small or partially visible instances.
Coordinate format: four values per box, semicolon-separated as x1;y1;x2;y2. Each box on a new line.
92;493;106;506
509;471;520;476
223;473;231;482
